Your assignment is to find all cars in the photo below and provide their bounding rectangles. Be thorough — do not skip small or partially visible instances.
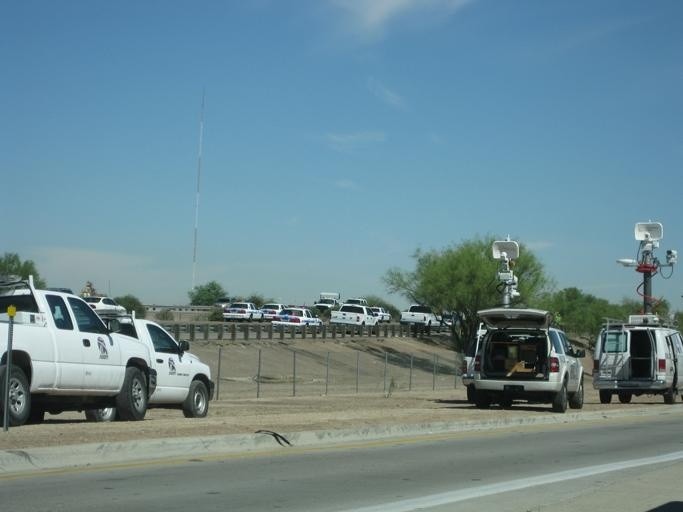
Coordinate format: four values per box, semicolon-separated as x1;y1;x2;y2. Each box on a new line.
79;293;127;315
215;291;453;334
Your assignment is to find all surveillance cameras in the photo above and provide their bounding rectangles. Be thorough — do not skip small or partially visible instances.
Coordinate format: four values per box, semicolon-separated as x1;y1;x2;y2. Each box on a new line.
667;249;677;256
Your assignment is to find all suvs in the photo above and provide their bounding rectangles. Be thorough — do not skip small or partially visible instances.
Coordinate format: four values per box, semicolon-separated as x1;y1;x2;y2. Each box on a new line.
470;306;587;415
44;287;72;293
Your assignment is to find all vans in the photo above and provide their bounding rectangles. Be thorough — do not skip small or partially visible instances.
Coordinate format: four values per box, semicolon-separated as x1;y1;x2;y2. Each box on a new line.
590;310;683;406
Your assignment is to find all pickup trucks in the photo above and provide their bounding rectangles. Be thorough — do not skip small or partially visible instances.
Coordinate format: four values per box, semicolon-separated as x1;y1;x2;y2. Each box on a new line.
83;310;216;422
0;273;157;427
460;321;487;406
